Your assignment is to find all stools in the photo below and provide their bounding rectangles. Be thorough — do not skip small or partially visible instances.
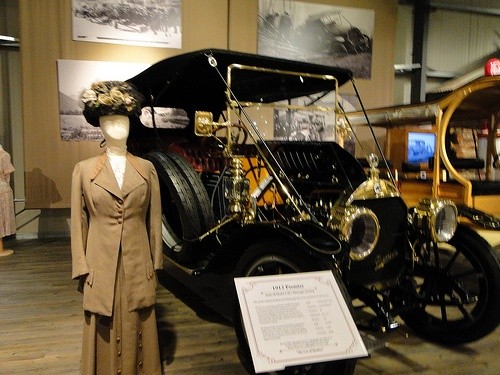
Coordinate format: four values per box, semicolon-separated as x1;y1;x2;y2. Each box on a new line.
427;155;483;182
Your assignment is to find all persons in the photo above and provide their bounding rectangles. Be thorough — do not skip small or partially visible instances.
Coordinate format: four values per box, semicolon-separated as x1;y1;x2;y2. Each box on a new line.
71;80;163;374
0;144;17;257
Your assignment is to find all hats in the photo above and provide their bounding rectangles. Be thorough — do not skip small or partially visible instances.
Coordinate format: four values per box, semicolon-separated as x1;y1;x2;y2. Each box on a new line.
80;80;142;127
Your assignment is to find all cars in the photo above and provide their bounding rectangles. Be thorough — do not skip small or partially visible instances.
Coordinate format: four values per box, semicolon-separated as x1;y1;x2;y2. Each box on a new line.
122;48;500;374
